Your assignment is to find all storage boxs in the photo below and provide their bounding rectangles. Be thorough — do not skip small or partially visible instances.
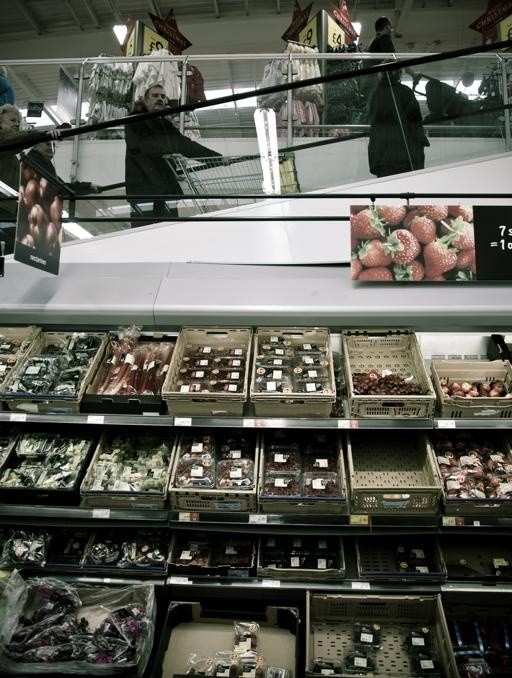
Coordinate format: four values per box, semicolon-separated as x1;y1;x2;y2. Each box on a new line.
2;584;155;678
161;325;253;416
0;329;110;414
167;531;258;579
0;326;42;392
258;429;348;513
79;333;178;414
0;432;98;507
250;327;336;418
487;333;512;364
152;600;302;678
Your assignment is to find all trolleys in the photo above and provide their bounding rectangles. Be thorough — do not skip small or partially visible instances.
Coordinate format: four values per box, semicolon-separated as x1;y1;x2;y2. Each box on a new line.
163;147;302;212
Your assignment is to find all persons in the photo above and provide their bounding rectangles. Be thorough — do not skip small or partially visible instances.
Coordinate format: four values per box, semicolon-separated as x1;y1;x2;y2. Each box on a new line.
364;15;420;82
367;59;431;179
25;140;103;197
0;66;15;106
124;84;224;229
1;103;69;259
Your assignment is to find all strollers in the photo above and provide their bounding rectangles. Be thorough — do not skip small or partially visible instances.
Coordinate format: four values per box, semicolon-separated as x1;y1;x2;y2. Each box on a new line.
414;73;504;136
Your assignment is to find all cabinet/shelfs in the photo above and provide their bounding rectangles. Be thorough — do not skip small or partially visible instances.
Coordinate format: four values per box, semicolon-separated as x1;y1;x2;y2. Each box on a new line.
0;323;512;678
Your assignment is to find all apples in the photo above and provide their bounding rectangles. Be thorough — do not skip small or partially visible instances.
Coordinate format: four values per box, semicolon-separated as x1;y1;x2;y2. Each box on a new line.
439;377;511;398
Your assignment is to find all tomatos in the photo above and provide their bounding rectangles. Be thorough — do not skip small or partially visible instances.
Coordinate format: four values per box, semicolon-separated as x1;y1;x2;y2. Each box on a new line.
16;165;62;259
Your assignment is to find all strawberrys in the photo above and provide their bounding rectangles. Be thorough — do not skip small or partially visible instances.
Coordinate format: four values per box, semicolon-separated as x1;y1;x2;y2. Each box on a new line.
351;206;475;282
